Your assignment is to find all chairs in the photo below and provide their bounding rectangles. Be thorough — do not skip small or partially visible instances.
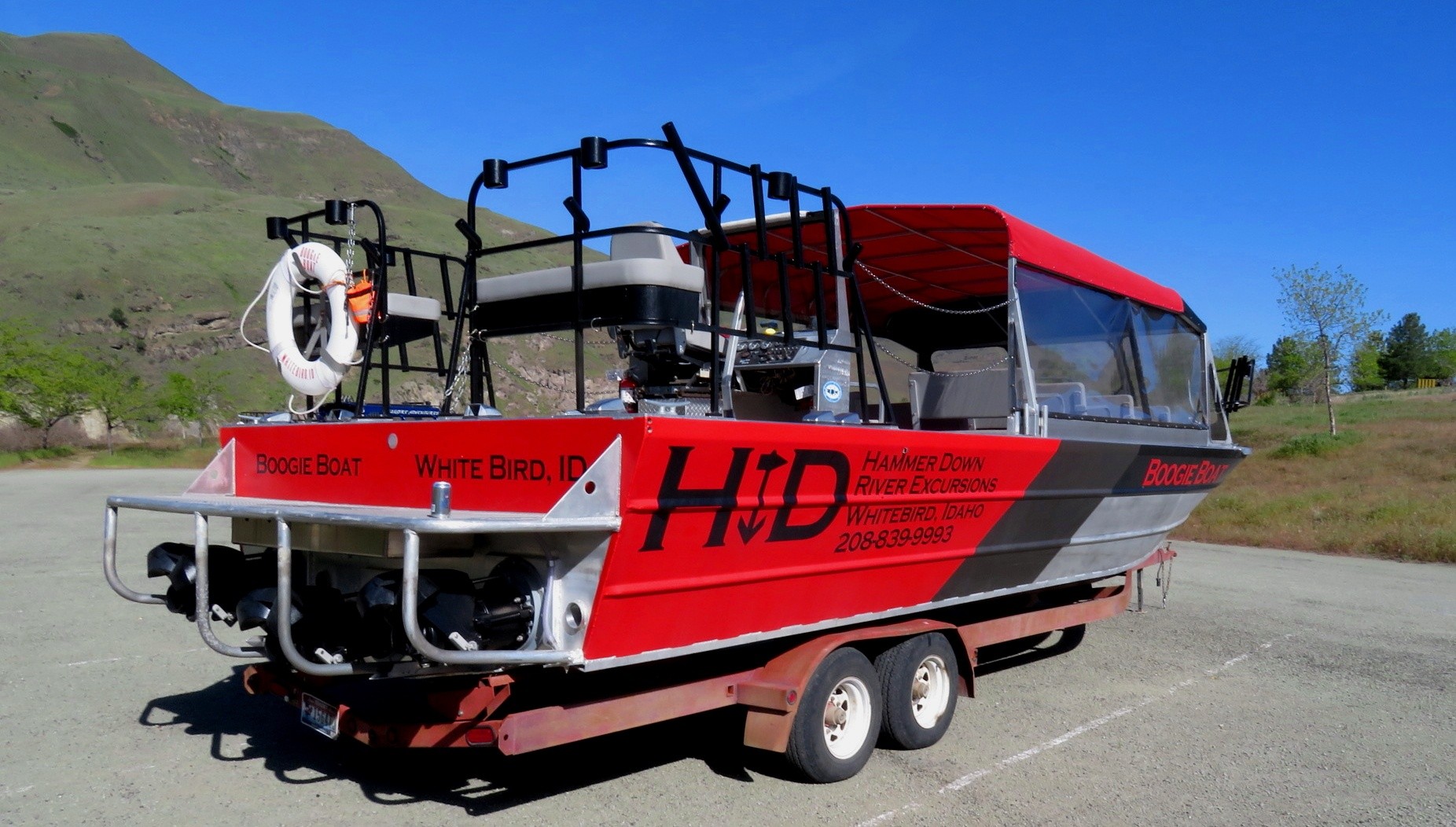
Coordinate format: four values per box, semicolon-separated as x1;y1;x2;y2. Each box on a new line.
1134;405;1172;422
611;220;707;334
849;391;864;421
1085;394;1135;419
1035;382;1089;416
908;367;1015;431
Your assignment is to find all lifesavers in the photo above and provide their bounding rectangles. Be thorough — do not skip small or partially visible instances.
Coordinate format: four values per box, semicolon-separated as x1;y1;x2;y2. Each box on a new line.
265;240;360;396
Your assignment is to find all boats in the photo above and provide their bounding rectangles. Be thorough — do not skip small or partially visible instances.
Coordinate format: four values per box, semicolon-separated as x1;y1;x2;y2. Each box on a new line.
104;123;1257;673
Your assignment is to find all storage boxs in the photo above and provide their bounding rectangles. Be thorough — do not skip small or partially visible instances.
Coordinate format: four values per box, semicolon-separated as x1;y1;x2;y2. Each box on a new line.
290;292;443;351
474;257;706;340
